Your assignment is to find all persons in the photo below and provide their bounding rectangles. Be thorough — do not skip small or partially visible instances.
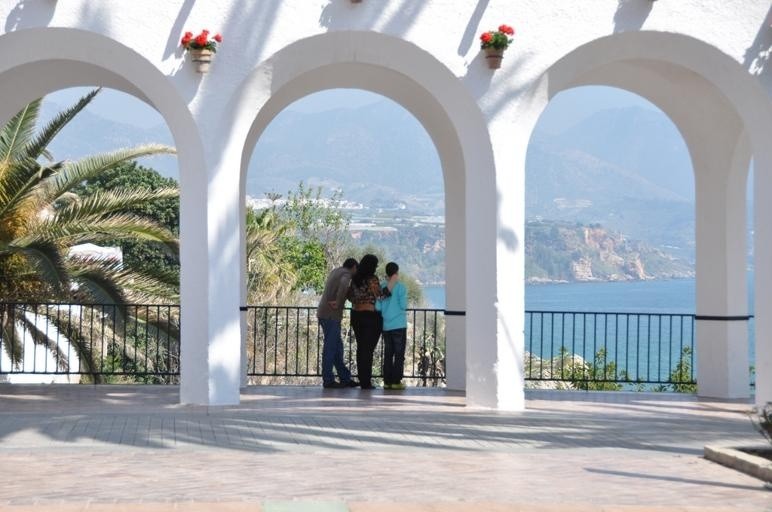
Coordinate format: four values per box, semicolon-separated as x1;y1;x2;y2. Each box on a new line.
316;257;359;387
374;262;407;389
347;254;398;390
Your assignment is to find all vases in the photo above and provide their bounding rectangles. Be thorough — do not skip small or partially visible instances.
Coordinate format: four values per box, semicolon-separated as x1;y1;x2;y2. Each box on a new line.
483;46;506;70
189;49;213;76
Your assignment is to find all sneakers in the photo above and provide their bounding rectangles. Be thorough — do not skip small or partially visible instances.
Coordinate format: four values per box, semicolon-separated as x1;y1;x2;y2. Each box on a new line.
324;379;406;389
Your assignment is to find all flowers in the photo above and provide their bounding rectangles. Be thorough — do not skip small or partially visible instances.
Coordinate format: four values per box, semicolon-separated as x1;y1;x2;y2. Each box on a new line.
476;24;516;52
178;29;221;52
